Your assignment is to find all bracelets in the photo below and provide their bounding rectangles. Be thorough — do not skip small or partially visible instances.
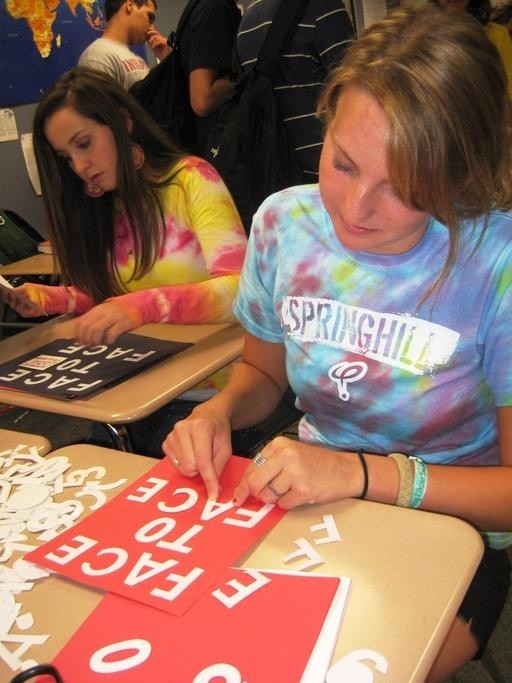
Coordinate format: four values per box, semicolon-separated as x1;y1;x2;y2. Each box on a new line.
410;457;428;510
388;452;413;507
355;447;372;501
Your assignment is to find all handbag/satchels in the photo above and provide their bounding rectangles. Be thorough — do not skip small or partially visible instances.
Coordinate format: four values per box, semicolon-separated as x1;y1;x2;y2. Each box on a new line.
207;1;307;237
127;0;219;153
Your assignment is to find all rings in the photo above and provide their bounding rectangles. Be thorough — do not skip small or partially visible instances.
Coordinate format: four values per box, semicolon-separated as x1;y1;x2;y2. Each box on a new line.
249;450;268;467
170;454;184;467
267;481;284;499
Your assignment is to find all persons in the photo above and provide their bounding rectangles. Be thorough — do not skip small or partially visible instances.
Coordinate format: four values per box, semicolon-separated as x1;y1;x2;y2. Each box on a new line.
387;2;512;238
173;2;246;158
232;2;355;183
78;2;174;94
2;68;247;459
162;2;512;681
